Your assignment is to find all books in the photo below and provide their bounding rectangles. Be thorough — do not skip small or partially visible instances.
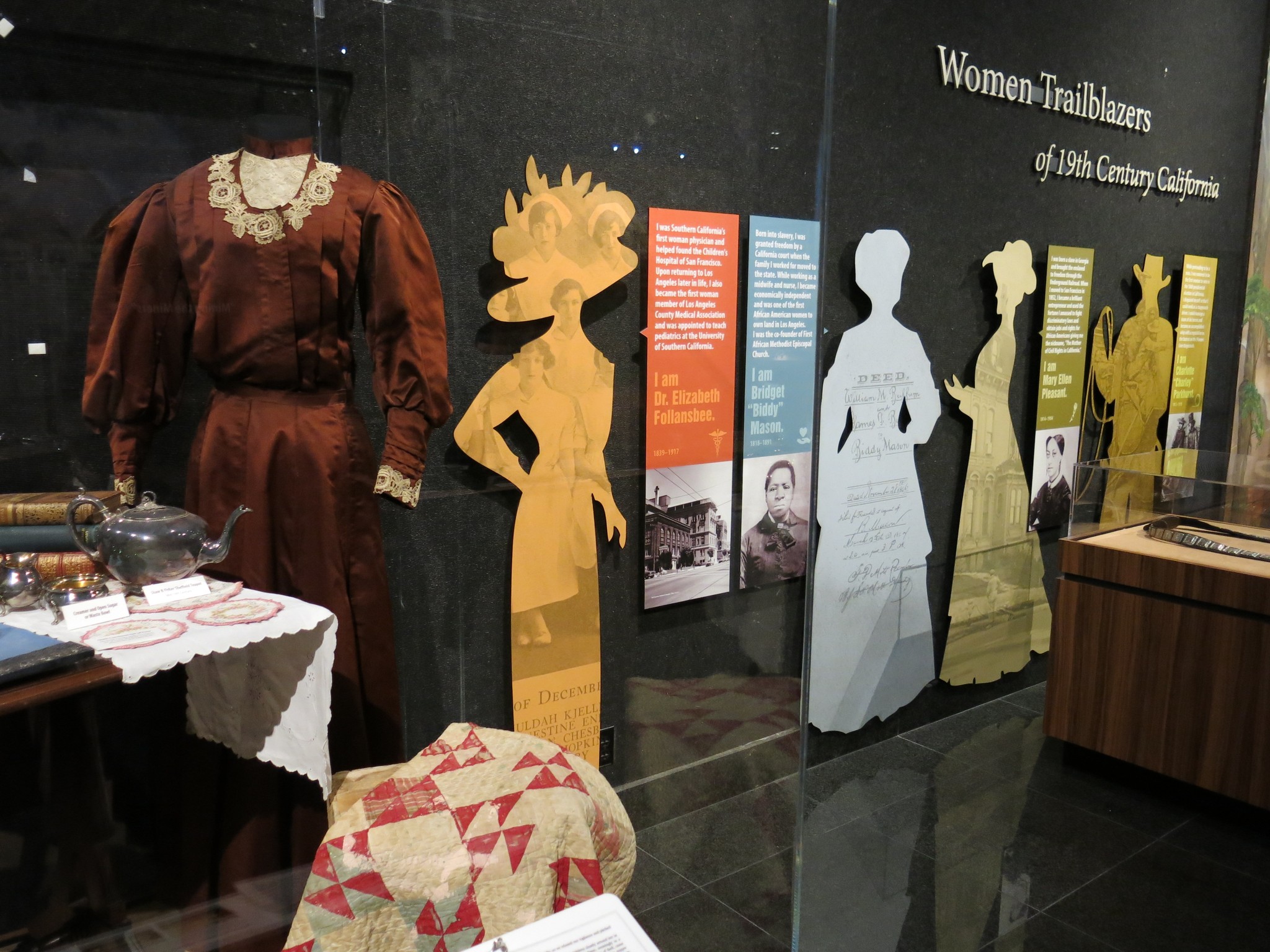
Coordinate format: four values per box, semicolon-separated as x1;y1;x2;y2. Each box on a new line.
0;554;112;577
0;525;99;553
0;490;126;526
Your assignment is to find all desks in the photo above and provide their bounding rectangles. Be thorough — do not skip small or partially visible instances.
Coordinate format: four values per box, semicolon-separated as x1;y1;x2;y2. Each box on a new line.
0;574;344;952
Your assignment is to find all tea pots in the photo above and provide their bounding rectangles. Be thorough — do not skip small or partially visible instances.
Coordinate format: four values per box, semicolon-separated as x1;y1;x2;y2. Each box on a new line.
66;491;254;585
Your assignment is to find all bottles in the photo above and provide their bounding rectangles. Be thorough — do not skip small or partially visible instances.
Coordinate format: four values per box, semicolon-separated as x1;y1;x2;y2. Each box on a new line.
0;552;42;616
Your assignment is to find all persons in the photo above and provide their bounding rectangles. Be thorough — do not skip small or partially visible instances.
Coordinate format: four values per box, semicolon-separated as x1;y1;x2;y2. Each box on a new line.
1172;418;1186;448
83;149;454;952
739;460;809;589
1029;435;1071;529
1187;413;1199;450
